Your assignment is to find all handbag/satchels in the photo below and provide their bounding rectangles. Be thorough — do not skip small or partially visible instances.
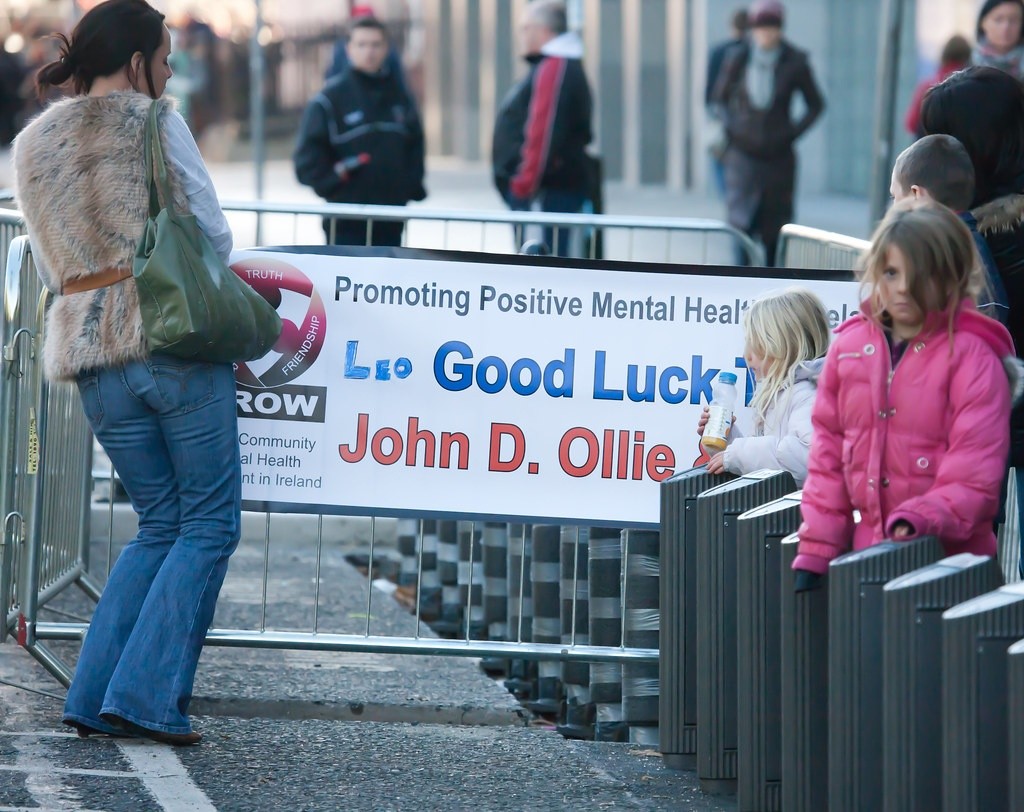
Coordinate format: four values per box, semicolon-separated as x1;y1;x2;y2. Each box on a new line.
130;100;283;360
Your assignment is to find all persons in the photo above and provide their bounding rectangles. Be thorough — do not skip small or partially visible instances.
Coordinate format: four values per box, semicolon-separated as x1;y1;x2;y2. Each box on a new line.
921;64;1024;471
696;291;829;490
293;0;426;247
888;132;1003;321
1;0;240;746
490;3;604;261
791;198;1024;595
706;2;825;269
904;1;1024;137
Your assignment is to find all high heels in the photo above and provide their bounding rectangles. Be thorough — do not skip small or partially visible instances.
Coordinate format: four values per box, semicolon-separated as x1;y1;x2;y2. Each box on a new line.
75;721;121;737
107;712;202;743
